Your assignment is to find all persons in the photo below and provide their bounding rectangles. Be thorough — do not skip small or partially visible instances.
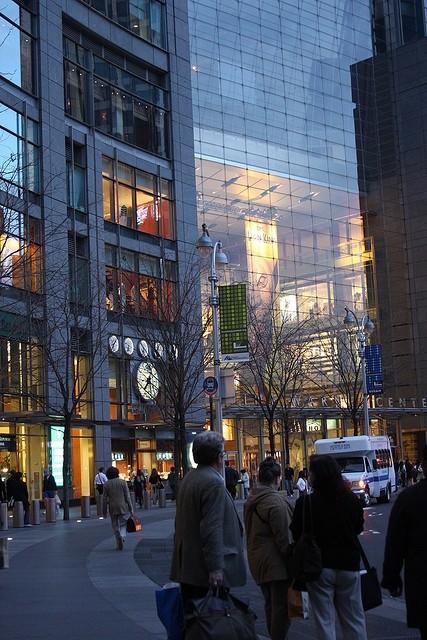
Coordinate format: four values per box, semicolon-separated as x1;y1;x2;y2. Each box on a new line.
239;469;251;500
94;466;107;496
289;455;366;640
136;470;147;509
14;471;34;527
224;460;239;500
381;453;426;640
394;460;400;488
168;430;246;600
404;458;415;487
167;465;178;502
283;461;296;498
297;467;309;497
6;468;17;502
243;456;295;639
45;475;59;522
147;467;162;505
102;467;135;551
398;458;405;488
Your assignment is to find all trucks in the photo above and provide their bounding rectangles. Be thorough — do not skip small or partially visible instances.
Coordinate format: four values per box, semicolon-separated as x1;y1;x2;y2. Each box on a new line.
315;435;397;506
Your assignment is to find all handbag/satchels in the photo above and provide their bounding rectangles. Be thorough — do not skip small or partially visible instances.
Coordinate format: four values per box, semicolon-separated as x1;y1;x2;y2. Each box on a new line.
155;587;184;640
360;565;382;611
287;579;309;621
127;515;142;533
183;583;260;640
291;536;322;582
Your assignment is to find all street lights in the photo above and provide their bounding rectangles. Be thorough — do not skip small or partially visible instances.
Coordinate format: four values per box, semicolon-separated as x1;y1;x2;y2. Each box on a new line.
344;308;375;435
196;222;228;489
